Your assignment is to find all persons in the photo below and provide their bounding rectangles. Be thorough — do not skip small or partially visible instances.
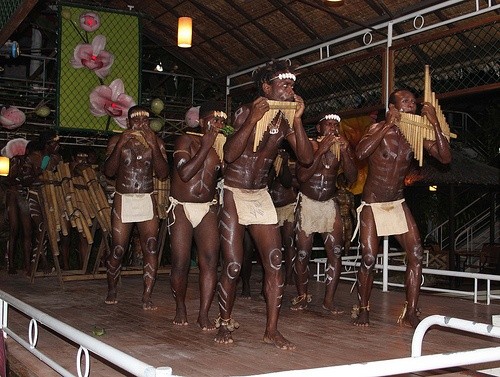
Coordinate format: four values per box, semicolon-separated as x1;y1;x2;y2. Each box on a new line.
215;62;315;350
352;88;453;327
291;110;356;314
170;103;230;331
0;103;296;308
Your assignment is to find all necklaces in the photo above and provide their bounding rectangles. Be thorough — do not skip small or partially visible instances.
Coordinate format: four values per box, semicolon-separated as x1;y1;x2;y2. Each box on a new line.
320;158;332;169
269;113;284;134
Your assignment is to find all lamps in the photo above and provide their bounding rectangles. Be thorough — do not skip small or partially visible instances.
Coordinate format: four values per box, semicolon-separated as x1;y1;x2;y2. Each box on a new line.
177;17;193;48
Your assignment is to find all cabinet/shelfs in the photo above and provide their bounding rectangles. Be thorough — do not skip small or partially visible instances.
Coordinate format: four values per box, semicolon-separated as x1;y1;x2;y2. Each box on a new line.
0;52;193;148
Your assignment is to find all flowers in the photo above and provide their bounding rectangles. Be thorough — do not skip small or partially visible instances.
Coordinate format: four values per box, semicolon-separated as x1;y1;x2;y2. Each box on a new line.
60;10;137;129
